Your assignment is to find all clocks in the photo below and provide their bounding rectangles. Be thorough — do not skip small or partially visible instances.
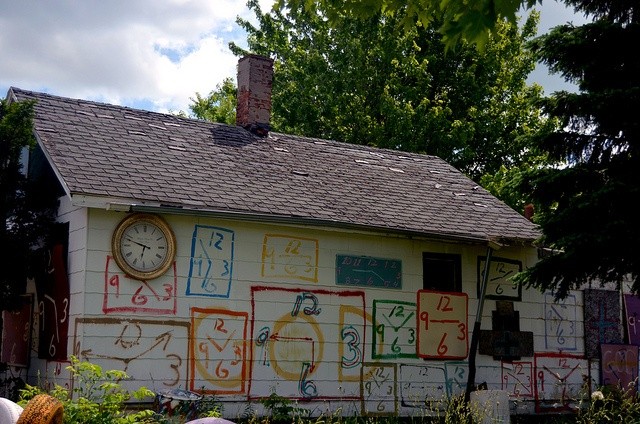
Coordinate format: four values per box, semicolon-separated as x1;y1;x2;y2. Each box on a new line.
111;212;177;281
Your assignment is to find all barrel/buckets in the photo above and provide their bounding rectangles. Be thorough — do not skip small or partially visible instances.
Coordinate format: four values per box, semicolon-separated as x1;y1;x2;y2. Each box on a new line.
470;389;511;424
153;389;202;421
